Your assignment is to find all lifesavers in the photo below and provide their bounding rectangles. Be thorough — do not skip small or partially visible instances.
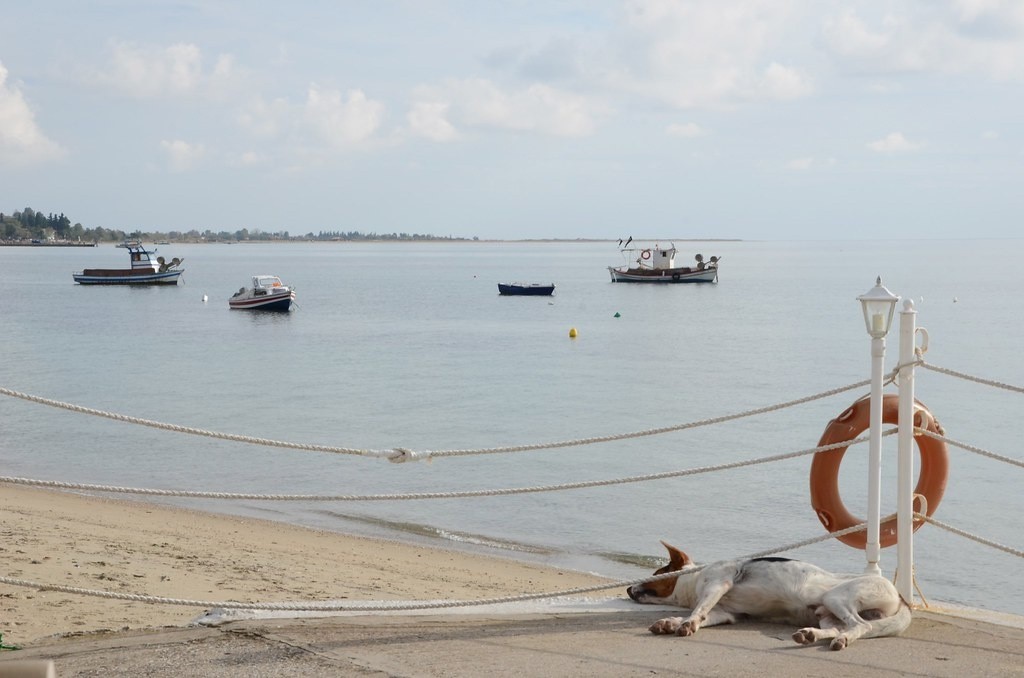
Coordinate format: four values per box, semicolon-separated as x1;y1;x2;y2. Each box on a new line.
809;393;948;549
642;250;650;260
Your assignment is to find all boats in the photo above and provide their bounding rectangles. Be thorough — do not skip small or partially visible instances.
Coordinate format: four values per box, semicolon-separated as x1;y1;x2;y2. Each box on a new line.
607;235;721;282
229;275;296;311
498;282;557;295
154;240;170;245
116;236;142;248
72;244;185;286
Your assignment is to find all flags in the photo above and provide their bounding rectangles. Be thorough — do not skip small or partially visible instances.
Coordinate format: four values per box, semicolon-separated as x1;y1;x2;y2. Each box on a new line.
624;236;632;247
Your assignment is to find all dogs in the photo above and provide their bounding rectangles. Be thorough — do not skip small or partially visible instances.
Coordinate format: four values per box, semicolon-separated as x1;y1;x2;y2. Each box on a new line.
627;540;912;651
